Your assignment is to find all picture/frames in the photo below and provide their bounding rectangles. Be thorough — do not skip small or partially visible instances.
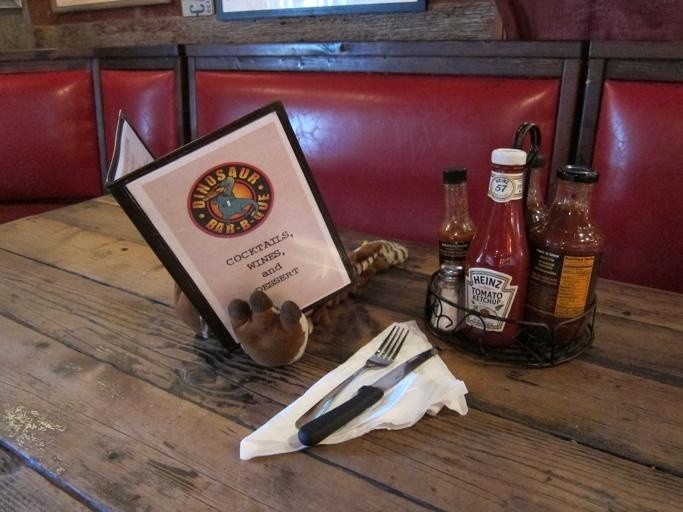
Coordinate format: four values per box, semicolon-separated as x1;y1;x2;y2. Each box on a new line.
49;0;172;14
215;1;426;21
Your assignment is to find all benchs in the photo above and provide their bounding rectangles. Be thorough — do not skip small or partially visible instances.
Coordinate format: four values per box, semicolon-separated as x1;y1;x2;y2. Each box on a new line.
182;41;582;249
0;47;182;226
571;40;683;293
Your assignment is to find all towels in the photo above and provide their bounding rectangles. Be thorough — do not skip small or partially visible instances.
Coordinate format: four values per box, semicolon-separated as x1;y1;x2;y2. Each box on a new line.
239;319;469;462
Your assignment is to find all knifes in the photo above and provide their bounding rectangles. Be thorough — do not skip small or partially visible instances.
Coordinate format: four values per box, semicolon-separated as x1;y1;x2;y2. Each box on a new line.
298;344;448;448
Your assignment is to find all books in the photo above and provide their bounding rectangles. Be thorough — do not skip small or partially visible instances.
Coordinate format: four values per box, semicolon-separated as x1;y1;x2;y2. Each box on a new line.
102;98;359;354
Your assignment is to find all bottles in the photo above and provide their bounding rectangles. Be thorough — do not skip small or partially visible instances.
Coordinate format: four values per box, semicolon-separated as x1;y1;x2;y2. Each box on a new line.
430;264;467;338
464;148;531;346
523;155;550;247
524;164;604;346
436;167;476;267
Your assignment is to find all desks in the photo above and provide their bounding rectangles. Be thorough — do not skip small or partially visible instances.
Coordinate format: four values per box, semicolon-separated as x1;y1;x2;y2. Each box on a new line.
0;195;683;512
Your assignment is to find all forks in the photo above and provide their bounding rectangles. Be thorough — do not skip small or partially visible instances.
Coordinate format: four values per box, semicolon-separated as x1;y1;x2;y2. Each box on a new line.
295;324;410;430
